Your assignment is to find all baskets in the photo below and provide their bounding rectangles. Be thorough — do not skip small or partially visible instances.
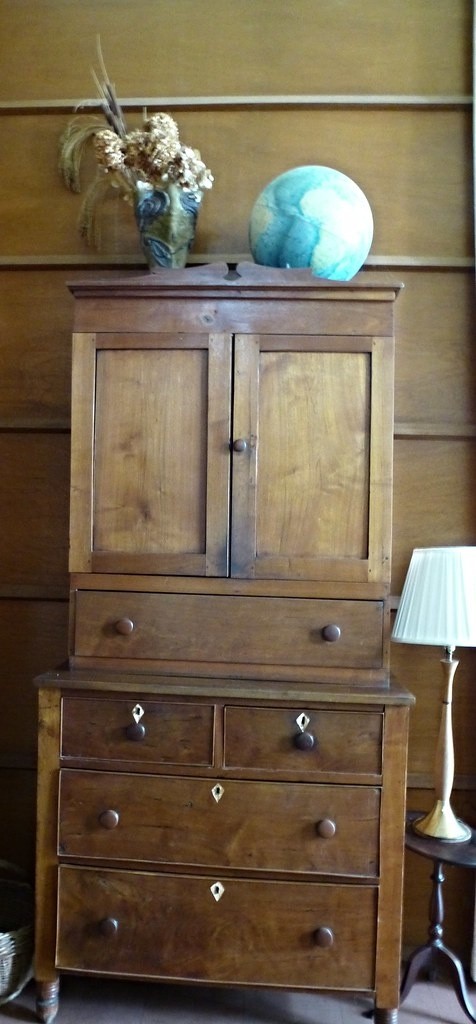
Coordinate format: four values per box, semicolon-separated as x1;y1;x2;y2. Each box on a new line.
0;859;35;1005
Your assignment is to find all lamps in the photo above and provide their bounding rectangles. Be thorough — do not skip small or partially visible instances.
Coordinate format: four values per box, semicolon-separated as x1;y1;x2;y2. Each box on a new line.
390;546;476;844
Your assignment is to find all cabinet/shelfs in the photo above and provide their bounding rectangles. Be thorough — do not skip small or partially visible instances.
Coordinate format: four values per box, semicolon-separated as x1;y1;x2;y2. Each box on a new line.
64;278;404;688
32;665;417;1024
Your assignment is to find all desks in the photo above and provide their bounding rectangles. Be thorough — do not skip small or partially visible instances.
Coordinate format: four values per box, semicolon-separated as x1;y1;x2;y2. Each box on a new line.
366;830;476;1024
